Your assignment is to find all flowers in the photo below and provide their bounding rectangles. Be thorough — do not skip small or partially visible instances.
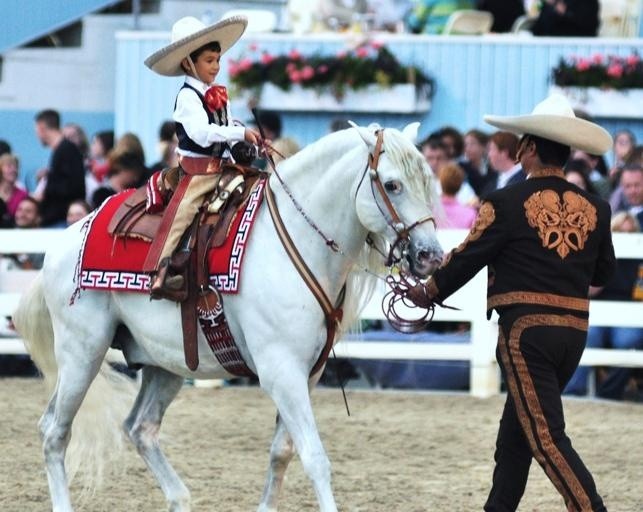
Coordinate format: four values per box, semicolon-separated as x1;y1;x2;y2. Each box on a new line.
226;38;439;96
551;52;642;92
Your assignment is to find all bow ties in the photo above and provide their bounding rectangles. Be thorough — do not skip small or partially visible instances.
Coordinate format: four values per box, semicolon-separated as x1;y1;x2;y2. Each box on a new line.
204;86;227;113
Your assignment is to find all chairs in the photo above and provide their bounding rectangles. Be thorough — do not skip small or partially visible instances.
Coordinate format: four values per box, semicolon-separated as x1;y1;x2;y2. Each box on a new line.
221;0;536;37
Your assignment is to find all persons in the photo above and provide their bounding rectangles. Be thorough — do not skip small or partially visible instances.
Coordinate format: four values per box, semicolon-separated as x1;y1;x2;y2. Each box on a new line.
407;92;615;512
145;15;248;299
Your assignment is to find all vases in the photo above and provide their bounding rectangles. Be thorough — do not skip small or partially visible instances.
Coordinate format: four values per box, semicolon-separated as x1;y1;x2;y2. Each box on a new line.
257;80;432;113
554;83;642;121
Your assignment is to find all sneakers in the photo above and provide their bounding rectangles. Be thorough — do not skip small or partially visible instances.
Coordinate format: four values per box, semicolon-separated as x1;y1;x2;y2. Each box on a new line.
150;274;183;301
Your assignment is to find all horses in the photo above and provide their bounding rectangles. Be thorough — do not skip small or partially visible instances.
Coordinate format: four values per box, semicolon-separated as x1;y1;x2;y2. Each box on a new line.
10;119;444;512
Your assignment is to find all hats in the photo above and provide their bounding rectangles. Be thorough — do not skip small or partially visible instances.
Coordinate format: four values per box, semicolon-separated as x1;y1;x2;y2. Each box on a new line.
481;92;614;156
143;15;250;78
